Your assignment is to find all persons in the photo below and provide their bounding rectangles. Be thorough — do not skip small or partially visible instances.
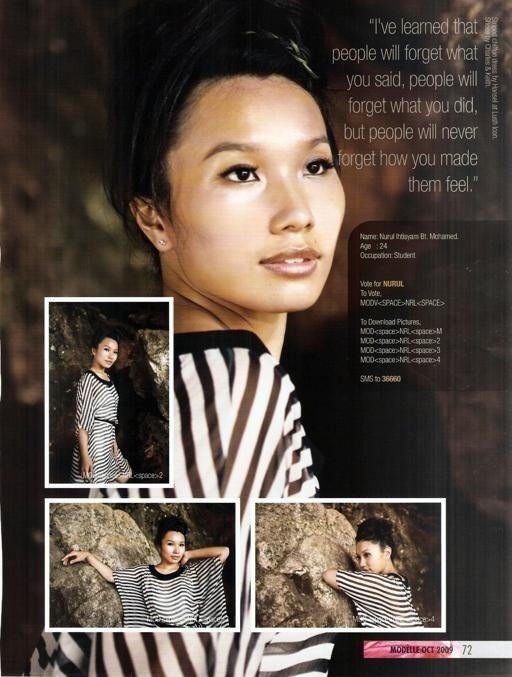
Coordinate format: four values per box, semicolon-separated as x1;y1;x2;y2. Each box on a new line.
61;513;231;628
72;329;133;482
321;516;425;628
25;1;348;675
